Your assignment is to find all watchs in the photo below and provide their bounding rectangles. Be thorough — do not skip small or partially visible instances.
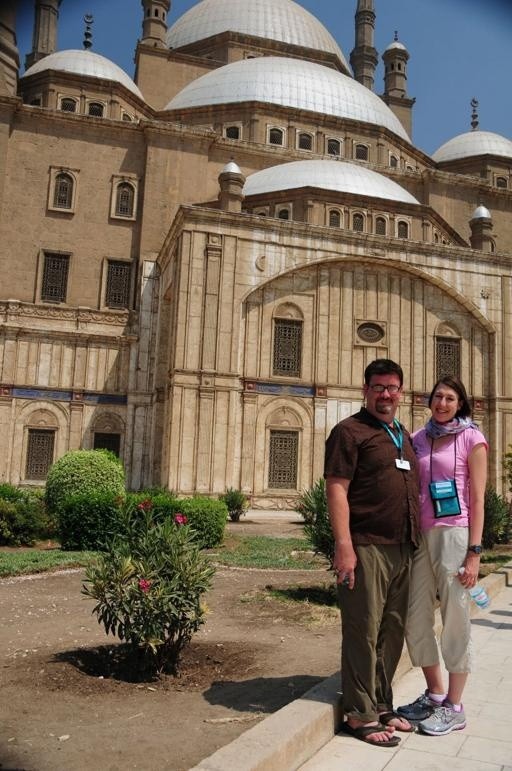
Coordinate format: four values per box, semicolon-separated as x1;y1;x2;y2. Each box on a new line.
469;545;482;554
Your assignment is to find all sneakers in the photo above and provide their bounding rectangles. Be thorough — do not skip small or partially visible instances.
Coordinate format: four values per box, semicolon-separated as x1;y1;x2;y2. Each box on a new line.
396;688;444;721
417;698;467;736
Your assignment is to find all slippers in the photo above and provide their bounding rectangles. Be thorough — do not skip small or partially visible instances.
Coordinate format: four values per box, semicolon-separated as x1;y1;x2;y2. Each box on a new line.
340;720;402;747
378;711;417;733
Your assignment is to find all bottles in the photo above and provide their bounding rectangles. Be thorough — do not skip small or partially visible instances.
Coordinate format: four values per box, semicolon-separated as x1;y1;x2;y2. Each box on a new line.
458;567;489;611
327;552;357;594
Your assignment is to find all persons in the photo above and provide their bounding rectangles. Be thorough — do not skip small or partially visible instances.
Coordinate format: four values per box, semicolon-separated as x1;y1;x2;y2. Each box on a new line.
398;373;487;735
323;358;420;748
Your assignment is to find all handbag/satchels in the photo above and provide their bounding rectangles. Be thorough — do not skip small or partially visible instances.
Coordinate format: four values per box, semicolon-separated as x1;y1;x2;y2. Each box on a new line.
428;480;461;517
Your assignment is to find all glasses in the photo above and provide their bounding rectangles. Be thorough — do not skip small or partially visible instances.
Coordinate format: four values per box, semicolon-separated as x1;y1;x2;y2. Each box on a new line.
365;383;403;394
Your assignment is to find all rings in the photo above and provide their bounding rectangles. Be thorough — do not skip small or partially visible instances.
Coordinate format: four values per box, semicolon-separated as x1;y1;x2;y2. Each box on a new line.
336;570;340;573
